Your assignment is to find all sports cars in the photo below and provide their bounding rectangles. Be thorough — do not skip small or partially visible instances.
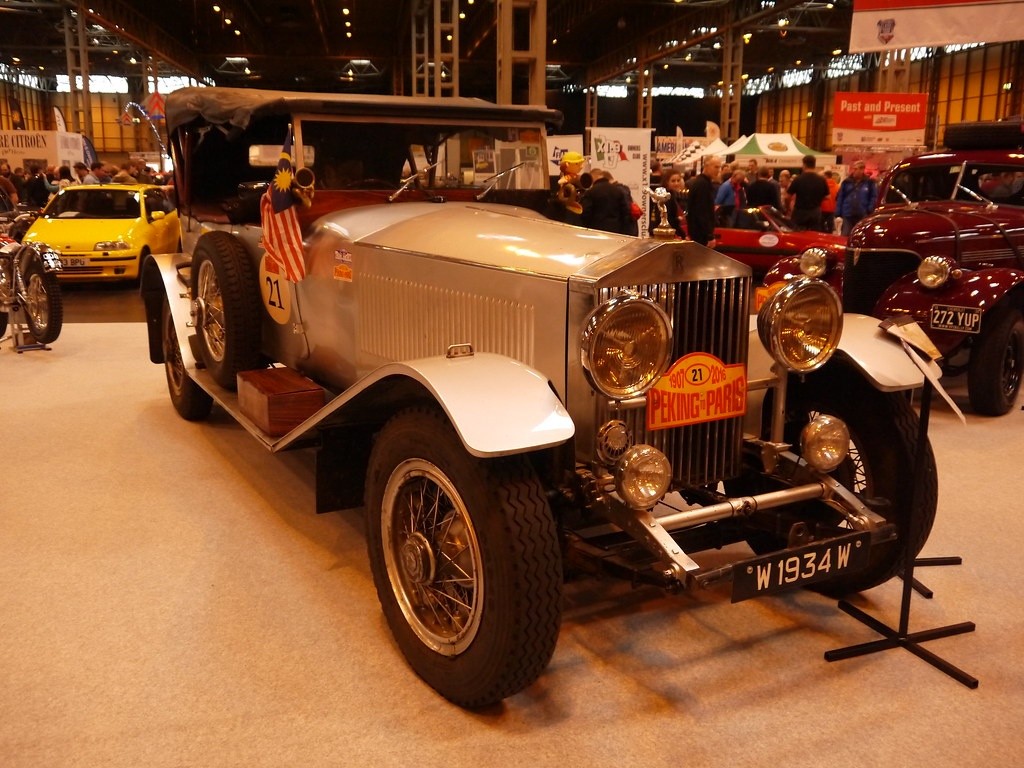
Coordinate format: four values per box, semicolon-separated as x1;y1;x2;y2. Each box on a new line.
673;204;849;287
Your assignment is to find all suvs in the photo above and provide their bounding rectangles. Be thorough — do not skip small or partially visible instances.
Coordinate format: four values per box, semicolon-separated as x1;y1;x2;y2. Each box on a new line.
141;87;964;708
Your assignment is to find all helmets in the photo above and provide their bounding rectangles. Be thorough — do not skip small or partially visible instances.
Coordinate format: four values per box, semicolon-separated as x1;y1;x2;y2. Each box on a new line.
561;152;585;163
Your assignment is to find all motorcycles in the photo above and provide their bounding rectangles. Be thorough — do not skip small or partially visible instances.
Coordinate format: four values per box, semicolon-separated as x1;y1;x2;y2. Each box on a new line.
0;218;64;355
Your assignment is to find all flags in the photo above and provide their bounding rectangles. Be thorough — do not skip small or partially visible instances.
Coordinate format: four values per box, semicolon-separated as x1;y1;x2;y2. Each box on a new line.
849;0;1024;54
260;130;306;283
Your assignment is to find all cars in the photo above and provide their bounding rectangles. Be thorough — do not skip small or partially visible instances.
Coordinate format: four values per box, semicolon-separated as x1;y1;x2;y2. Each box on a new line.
22;183;180;296
761;151;1024;418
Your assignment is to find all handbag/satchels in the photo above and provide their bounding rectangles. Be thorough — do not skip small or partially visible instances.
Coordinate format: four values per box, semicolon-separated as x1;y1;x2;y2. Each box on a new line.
674;225;688;239
631;203;643;221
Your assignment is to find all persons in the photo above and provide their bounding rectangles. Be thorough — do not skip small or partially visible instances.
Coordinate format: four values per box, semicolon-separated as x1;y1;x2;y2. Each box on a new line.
650;155;878;245
0;159;176;213
979;172;1024;197
577;169;639;236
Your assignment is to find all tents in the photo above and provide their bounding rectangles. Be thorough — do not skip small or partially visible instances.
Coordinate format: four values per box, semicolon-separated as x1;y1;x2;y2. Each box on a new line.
661;132;837;175
